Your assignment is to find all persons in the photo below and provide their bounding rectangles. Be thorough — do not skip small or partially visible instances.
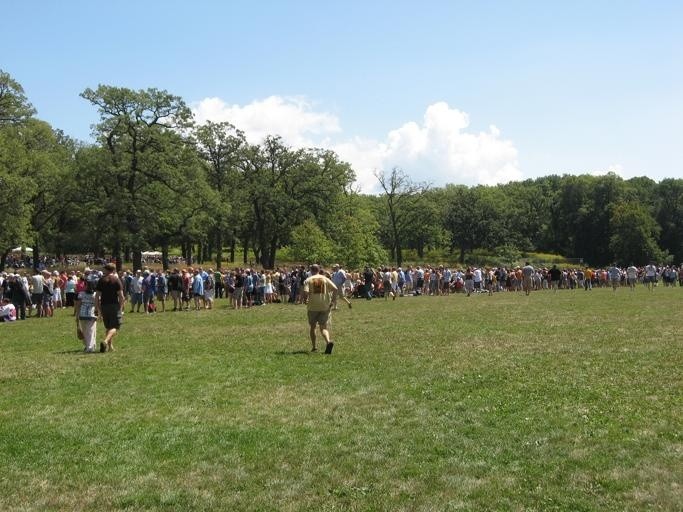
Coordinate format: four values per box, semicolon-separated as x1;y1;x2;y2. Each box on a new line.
94;263;125;353
1;255;682;321
302;263;338;354
75;279;99;352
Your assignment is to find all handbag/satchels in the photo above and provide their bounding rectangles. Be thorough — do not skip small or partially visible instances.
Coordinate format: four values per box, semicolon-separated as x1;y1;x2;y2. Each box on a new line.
76;329;83;339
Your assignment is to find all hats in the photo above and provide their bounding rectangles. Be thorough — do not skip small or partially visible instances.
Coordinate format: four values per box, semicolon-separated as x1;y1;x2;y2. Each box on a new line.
103;263;117;270
309;264;320;272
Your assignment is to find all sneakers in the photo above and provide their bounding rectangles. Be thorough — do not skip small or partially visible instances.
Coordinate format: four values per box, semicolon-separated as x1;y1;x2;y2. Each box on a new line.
100;342;107;352
311;348;318;352
325;343;333;354
